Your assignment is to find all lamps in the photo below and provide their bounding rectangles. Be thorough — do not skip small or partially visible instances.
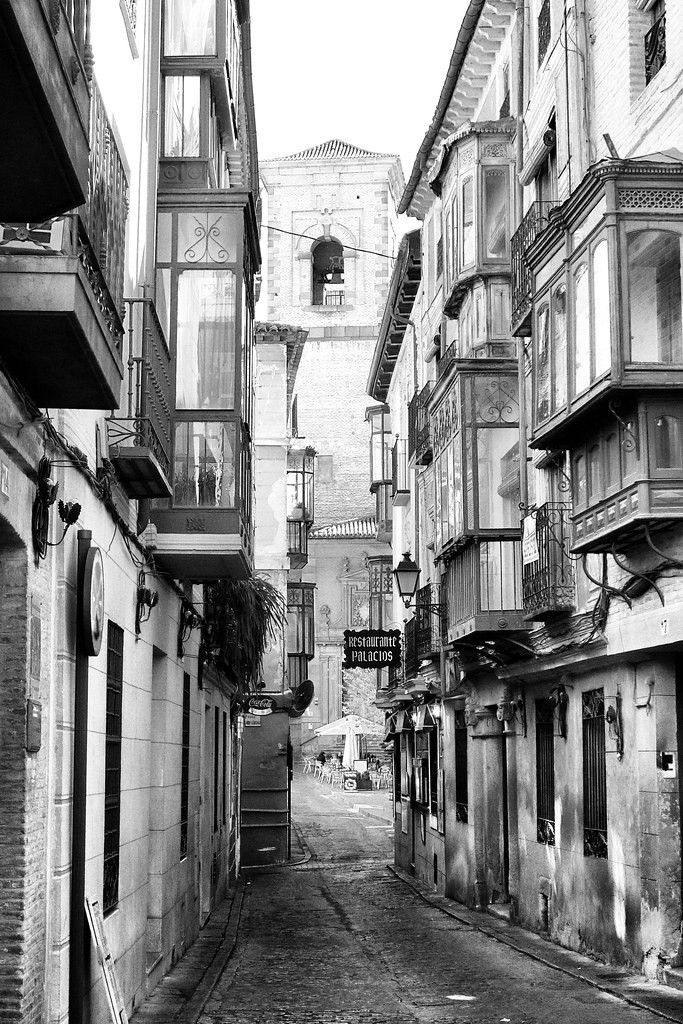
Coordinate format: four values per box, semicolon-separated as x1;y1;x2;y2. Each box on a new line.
392;550;447;619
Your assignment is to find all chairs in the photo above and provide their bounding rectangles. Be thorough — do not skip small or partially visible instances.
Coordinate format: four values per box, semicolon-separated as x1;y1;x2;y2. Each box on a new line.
302;754;380;790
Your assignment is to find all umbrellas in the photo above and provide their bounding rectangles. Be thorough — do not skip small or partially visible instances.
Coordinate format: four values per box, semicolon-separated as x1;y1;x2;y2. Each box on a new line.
313;715;386;758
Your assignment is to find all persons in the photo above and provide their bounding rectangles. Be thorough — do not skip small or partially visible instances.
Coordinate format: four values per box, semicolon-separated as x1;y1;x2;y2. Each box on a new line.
317;750;376;767
